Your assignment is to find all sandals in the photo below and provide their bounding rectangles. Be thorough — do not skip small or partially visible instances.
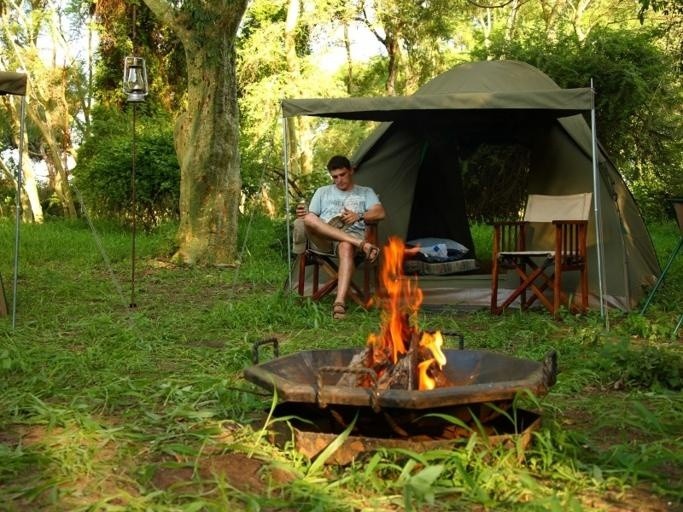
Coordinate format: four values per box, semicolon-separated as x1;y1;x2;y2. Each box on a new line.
332;301;348;322
359;239;380;265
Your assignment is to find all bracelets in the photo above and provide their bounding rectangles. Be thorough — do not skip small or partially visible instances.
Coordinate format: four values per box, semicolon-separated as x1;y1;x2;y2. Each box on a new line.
356;211;364;223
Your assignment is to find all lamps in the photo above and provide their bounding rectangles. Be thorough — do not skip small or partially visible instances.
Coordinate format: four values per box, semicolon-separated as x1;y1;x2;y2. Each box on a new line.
122;47;149;103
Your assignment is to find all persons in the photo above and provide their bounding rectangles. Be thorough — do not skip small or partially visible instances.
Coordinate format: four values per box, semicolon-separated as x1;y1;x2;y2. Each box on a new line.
295;155;385;321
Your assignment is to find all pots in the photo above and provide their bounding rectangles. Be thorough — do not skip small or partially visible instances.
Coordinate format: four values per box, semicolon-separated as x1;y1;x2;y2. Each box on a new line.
243;329;560;432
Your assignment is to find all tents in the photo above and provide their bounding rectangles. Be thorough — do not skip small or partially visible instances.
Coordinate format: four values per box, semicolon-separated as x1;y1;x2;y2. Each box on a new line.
278;53;665;313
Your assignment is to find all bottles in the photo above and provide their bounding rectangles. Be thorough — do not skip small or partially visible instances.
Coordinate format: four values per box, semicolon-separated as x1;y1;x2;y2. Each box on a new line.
298;201;309;215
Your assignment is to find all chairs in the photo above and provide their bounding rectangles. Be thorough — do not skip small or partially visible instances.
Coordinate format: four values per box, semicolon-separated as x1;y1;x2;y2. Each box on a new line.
491;192;592;322
299;194;382;311
